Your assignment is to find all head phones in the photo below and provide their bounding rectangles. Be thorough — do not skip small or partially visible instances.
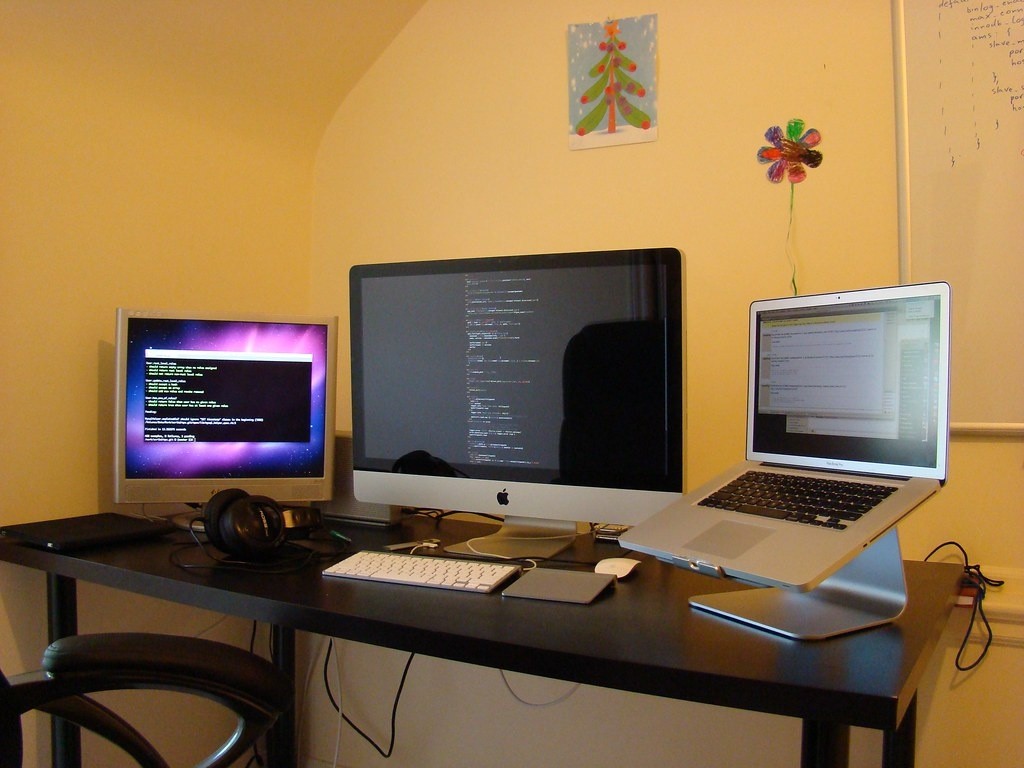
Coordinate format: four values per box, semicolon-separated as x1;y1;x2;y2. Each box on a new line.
200;488;311;556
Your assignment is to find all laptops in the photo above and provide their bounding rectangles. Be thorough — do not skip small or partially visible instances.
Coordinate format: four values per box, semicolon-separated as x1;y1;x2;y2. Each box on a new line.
616;285;952;590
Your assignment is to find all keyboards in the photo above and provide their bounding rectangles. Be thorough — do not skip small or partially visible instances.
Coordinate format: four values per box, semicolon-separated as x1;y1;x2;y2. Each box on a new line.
321;549;522;593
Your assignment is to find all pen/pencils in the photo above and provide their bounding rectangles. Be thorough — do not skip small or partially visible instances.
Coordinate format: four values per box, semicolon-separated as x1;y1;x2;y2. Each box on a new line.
329;530;352;542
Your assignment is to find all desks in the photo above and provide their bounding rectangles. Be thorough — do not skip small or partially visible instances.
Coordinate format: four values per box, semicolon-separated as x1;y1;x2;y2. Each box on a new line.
0;501;966;768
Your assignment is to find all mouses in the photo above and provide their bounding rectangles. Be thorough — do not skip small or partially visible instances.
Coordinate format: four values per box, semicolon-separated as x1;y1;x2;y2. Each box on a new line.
594;557;641;579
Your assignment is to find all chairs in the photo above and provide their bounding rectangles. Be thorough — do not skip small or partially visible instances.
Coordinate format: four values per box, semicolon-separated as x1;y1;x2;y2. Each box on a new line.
0;631;288;768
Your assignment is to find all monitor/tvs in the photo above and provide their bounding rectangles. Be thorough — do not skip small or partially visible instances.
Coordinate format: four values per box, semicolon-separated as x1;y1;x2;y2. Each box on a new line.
348;248;685;527
112;308;338;503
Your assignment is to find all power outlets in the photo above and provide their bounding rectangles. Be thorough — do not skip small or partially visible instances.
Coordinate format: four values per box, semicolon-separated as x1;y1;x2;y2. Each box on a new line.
954;562;980;609
